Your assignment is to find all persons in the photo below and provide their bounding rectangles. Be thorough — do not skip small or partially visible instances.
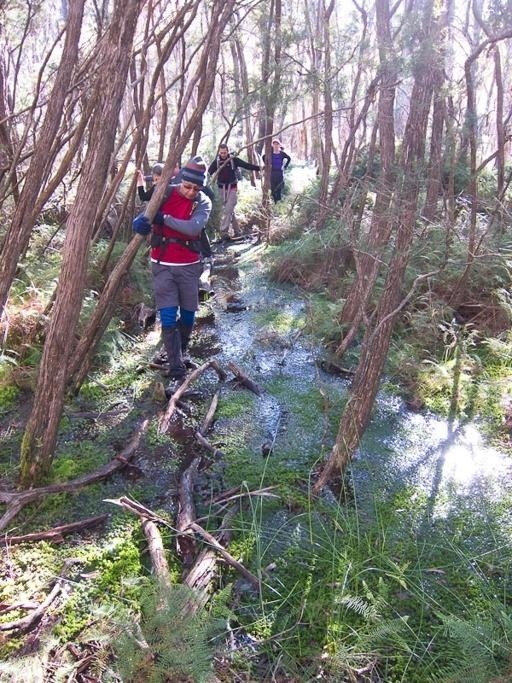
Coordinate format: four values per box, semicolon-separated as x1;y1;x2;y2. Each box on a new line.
208;144;272;241
262;139;291;204
131;154;214;399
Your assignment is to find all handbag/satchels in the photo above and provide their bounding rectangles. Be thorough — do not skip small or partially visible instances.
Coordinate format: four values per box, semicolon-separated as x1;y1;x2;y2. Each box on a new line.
190;228;211;258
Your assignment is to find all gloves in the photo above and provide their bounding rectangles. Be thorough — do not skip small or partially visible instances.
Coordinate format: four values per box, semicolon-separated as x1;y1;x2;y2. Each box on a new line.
132;211;165;236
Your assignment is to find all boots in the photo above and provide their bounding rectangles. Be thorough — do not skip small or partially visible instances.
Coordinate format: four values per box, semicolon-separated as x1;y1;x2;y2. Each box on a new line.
161;319;194;397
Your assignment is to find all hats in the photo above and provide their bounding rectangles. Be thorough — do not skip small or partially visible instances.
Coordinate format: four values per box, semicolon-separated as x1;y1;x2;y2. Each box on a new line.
180;155;206;186
